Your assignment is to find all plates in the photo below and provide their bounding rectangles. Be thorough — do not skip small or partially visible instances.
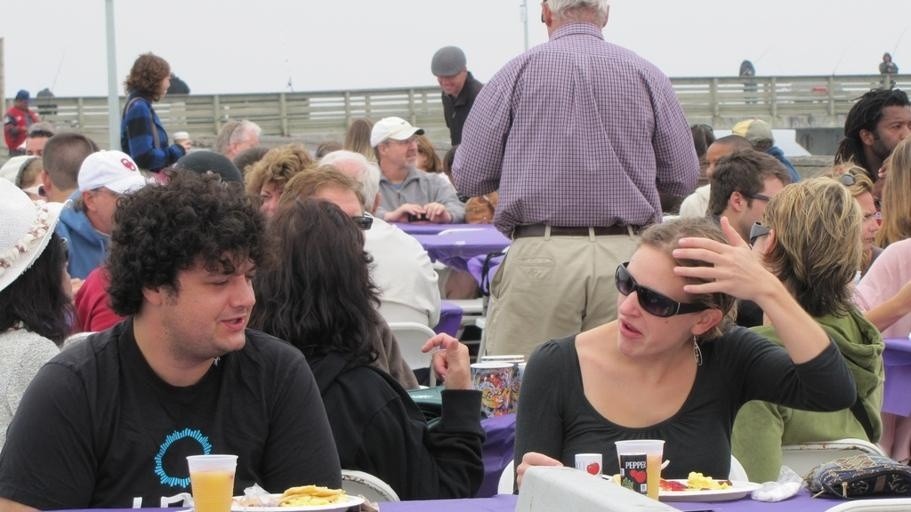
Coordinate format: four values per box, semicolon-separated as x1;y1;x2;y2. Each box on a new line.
233;494;365;511
660;478;759;502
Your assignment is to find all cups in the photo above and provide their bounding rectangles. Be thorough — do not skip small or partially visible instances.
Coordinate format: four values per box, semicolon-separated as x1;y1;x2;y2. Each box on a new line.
614;439;665;501
482;355;524;413
574;454;603;477
471;362;513;416
186;455;240;512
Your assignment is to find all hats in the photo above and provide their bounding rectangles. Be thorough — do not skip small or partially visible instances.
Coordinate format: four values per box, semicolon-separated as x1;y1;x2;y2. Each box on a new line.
731;119;773;144
370;117;424;147
432;46;466;76
0;177;73;291
78;150;156;196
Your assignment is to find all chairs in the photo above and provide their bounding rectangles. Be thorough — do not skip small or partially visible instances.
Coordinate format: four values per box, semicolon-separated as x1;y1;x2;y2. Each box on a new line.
341;469;400;500
781;438;888;478
386;322;442;388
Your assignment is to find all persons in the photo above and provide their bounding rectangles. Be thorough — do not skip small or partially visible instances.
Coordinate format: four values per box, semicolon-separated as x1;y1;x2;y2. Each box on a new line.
0;170;343;512
247;194;485;500
730;175;886;483
450;0;703;356
1;178;78;453
514;215;857;499
1;87;911;392
431;46;485;147
850;137;911;462
119;50;192;170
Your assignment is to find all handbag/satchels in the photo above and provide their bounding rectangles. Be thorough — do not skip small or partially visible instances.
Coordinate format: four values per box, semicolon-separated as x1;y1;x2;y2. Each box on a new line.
805;453;911;500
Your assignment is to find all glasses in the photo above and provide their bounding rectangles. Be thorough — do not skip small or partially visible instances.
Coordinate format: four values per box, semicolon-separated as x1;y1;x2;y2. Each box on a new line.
840;169;861;188
745;192;775;204
616;262;713;316
750;221;773;239
350;216;373;231
56;237;70;263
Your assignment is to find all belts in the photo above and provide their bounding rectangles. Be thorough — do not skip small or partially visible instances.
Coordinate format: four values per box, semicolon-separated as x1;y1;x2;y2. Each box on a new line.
513;224;642;236
